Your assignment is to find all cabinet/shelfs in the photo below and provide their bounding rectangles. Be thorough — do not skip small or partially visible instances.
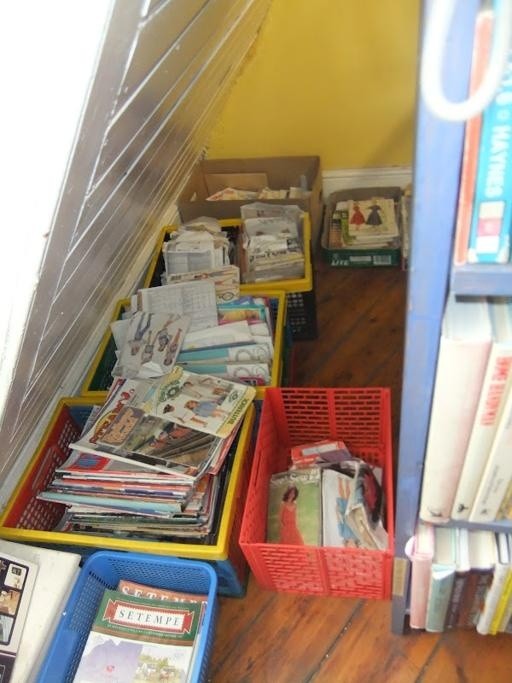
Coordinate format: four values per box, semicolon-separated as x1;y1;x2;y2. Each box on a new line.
390;1;511;636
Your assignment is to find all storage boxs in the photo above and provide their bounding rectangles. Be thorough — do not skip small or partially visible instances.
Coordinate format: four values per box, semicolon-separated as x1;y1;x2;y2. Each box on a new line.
176;157;324;254
81;290;289;418
36;551;218;683
145;211;319;341
238;385;394;601
320;184;405;268
0;396;253;598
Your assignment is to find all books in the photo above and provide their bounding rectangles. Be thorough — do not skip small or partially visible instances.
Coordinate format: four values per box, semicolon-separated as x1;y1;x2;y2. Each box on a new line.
451;7;511;270
266;440;388;554
144;223;306;292
327;198;400;250
205;185;314;201
73;578;207;683
420;295;512;527
0;552;38;683
109;293;273;387
37;365;256;544
407;528;512;634
